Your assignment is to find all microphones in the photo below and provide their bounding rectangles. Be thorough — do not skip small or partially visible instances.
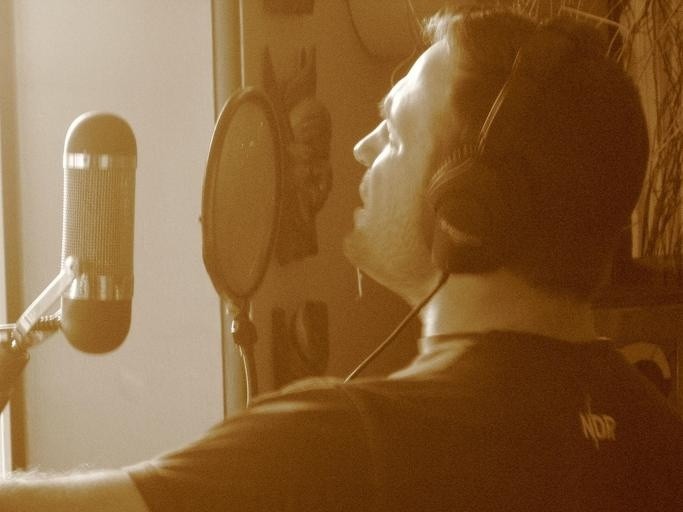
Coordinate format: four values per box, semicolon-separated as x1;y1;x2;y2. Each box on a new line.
60;112;137;356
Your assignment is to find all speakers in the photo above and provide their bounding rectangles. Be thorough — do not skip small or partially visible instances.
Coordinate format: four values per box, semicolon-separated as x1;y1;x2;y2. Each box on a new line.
593;265;682;411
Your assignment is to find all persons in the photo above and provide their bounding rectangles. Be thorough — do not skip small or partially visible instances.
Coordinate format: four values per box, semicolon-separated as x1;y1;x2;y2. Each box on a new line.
0;7;683;512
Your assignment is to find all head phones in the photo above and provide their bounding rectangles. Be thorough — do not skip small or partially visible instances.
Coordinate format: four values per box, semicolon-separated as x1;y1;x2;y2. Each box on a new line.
427;15;621;278
